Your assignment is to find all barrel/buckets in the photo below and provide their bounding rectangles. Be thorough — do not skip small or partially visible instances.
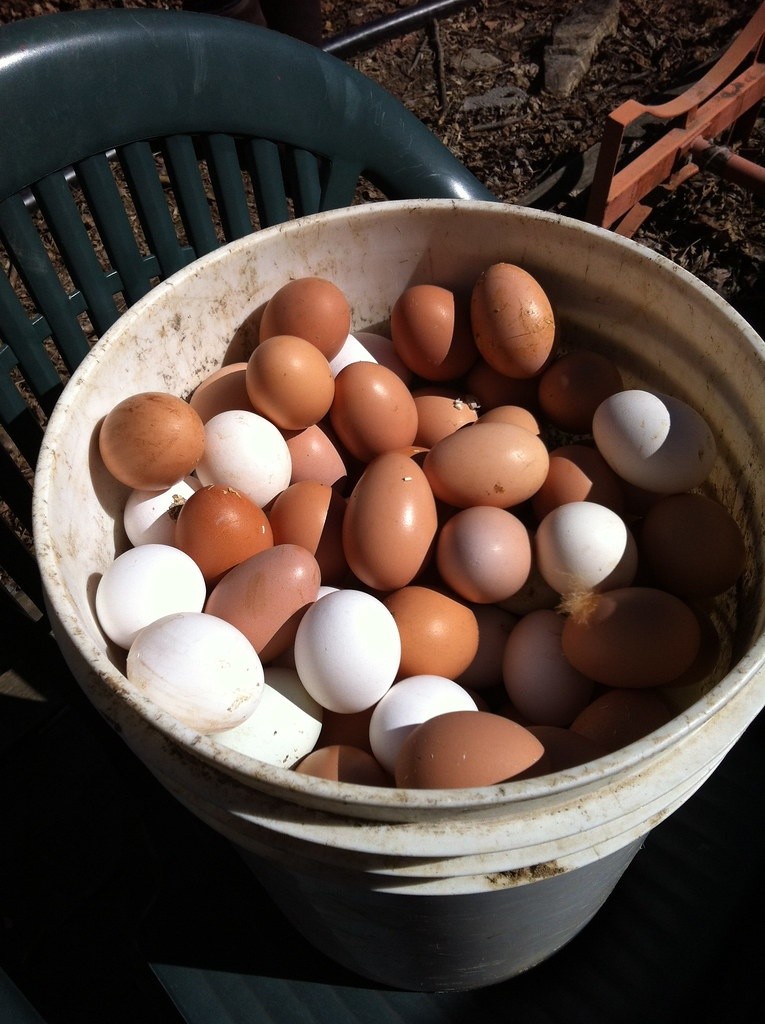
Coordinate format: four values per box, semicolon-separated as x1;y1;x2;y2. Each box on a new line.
30;196;764;994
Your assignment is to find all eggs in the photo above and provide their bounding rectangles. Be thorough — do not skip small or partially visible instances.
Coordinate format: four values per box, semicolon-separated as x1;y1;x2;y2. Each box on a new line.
97;259;746;795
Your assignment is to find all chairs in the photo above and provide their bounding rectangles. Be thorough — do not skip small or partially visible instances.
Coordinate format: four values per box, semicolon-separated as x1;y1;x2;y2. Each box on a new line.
0;8;765;1024
